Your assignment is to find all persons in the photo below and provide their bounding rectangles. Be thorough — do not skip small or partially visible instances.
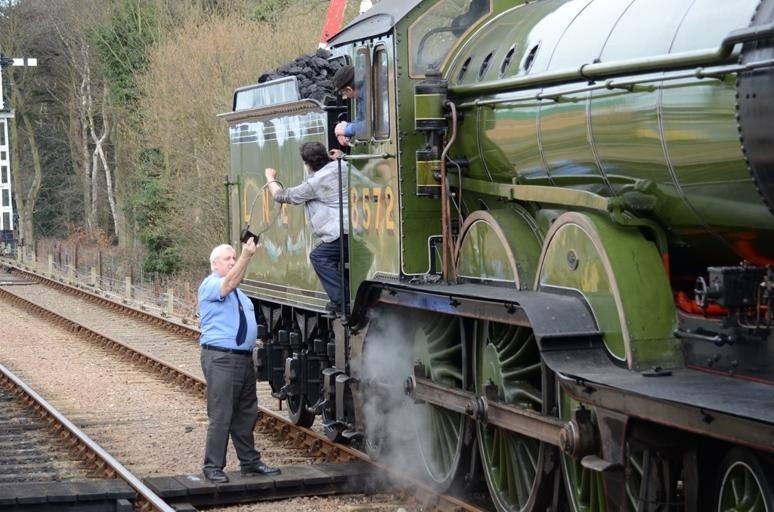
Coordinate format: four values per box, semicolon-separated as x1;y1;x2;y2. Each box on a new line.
197;236;282;484
333;65;367;146
264;142;350;316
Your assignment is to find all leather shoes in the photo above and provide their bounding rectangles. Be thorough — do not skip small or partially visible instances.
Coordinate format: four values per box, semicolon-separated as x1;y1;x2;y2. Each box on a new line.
239;463;281;476
325;302;348;314
205;470;230;483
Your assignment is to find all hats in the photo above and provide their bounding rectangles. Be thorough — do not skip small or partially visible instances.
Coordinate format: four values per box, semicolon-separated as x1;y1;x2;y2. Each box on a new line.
333;65;354;95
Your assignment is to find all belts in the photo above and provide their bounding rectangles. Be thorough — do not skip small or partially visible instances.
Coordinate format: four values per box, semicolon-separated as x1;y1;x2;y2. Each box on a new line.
203;344;254;357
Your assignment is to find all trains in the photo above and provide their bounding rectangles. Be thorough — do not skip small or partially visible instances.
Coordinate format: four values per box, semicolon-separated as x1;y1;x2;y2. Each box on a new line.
218;0;773;512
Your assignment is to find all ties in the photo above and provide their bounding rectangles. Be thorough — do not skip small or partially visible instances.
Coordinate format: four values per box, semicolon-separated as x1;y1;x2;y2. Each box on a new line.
234;288;247;346
